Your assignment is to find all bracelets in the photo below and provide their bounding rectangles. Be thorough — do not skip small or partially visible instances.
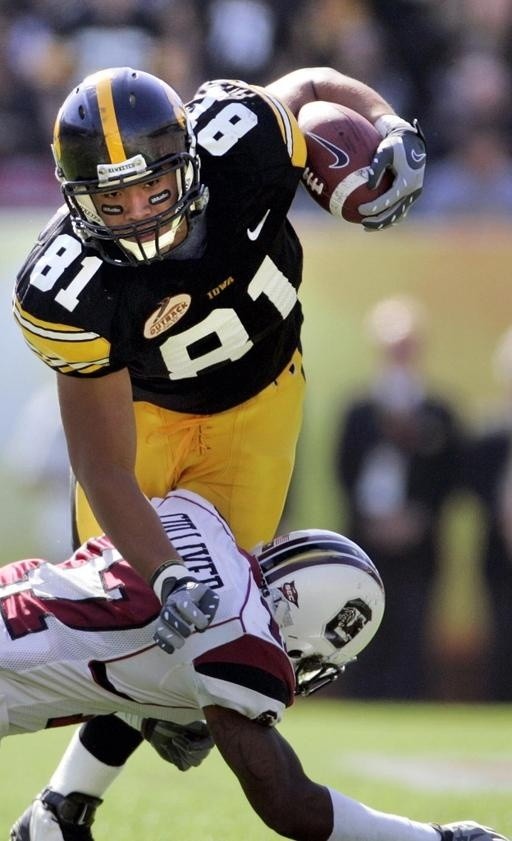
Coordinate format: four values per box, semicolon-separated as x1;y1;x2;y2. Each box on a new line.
151;560;193;603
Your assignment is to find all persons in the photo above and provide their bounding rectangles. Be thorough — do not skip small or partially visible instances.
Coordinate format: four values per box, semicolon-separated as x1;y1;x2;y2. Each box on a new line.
1;1;511;223
5;67;427;840
466;328;512;705
334;302;471;694
0;489;511;840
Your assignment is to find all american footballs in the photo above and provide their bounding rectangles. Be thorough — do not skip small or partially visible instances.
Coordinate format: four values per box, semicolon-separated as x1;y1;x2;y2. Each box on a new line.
298;101;395;223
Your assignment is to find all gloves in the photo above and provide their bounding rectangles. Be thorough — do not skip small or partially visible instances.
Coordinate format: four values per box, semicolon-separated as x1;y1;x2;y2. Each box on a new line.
140;718;211;772
432;819;506;841
148;559;218;653
358;119;428;231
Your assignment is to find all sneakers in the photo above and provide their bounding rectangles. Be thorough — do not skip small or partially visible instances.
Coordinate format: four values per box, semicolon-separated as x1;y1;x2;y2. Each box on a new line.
10;790;102;841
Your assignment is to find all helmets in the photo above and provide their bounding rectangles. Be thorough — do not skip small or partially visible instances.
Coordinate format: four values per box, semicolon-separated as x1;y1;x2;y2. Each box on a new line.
252;527;387;698
50;66;208;269
